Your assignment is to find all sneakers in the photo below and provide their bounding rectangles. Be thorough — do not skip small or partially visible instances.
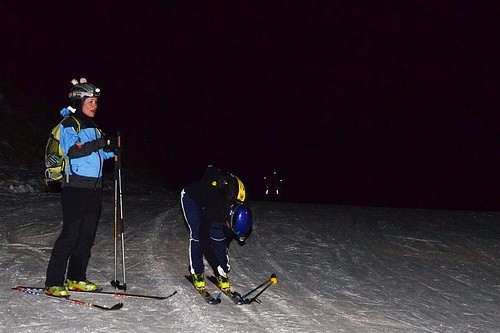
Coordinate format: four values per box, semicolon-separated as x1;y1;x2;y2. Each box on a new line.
66;278;97;291
44;285;68;297
213;270;230;290
192;271;207;290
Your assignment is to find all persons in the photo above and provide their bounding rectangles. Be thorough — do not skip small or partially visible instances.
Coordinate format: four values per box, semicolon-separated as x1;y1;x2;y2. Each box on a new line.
178;165;251;290
43;83;121;298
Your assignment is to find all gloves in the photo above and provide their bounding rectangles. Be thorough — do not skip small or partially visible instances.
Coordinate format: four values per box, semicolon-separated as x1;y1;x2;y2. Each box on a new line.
102;133;119;152
106;156;119;180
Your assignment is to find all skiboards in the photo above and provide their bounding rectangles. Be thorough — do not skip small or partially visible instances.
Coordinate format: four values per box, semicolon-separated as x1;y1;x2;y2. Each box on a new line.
11;285;177;310
184;274;245;305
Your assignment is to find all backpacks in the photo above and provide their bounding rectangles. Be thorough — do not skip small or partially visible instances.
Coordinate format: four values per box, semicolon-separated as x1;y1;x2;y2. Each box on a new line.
45;116;102;180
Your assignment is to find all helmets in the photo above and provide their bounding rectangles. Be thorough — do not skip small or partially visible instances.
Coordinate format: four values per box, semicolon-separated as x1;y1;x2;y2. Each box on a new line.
231;206;251;238
69;82;100;104
